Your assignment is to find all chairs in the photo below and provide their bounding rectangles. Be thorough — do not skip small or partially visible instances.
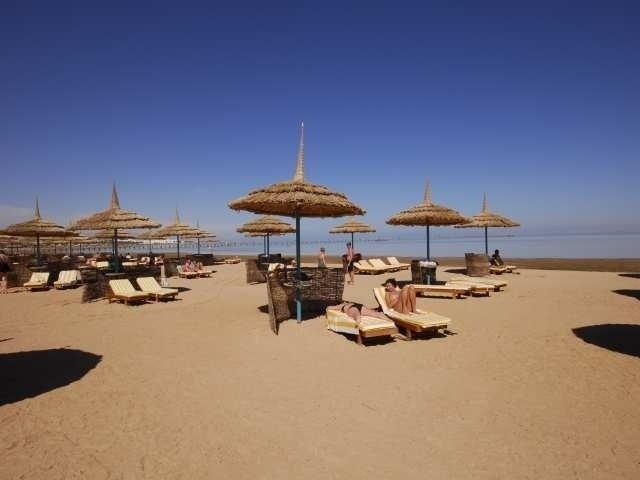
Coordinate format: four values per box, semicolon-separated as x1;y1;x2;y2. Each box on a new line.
325;256;517;345
23;264;212;306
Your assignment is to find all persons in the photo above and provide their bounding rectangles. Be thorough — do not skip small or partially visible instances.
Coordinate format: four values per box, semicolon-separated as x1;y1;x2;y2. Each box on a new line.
317;245;328;271
344;242;356;286
0;246;208;287
384;279;418;317
266;254;298;274
326;300;392;330
490;249;508;268
